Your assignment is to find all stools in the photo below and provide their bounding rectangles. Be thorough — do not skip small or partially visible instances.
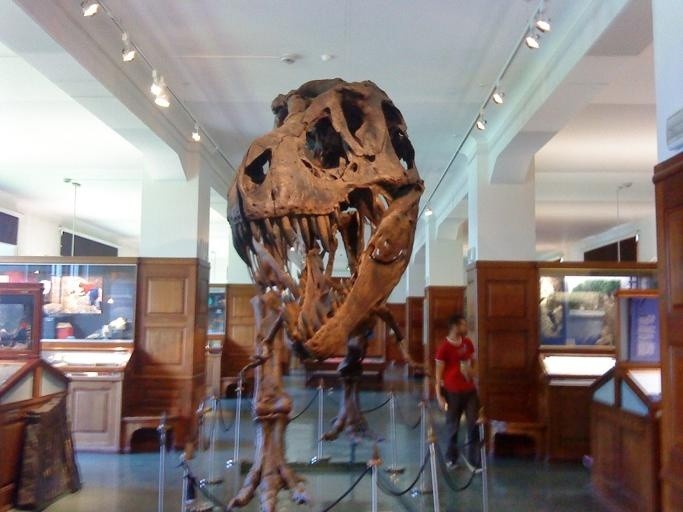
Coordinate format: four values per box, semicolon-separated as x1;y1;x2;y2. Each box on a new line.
487;411;545;461
122;406;186;454
221;373;255;399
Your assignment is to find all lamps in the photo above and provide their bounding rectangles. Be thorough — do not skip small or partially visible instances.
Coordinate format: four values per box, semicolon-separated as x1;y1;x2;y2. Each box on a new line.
524;3;552;51
78;0;100;19
475;79;506;132
120;30;170;110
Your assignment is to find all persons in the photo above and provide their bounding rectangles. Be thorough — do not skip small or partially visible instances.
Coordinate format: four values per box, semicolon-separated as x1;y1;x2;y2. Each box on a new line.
78;282;99;305
435;313;482;473
